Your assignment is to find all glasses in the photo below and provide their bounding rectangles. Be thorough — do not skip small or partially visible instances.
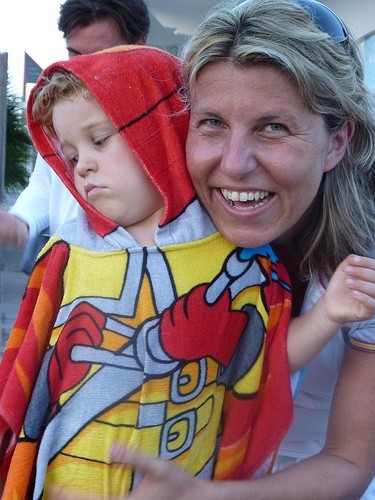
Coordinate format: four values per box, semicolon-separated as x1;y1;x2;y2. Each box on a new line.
290;0;359;78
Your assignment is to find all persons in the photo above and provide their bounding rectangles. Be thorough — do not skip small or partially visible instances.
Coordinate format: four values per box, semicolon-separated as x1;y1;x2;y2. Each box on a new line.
0;44;375;500
0;1;152;276
51;0;375;500
